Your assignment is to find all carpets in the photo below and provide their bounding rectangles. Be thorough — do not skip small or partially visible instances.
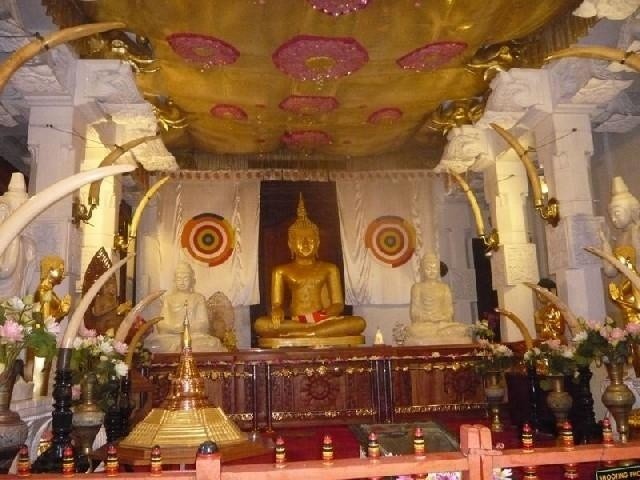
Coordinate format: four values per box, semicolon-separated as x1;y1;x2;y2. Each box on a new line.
347;418;460;480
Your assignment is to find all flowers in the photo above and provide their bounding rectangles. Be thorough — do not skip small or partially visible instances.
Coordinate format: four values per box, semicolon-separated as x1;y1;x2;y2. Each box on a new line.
0;296;59;372
70;323;131;385
626;316;640;375
523;339;579;377
572;322;626;368
466;316;516;374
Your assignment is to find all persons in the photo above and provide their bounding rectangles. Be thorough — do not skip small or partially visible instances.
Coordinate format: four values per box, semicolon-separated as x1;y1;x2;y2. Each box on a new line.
404;252;479;345
584;175;640;340
532;278;567;353
605;244;640;336
253;190;368;339
26;256;73;403
149;261;229;354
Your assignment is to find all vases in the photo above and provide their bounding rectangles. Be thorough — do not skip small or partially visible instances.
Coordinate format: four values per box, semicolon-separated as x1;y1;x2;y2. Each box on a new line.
70;381;105;459
600;364;637;440
484;370;509;432
0;366;28;477
548;377;573;433
